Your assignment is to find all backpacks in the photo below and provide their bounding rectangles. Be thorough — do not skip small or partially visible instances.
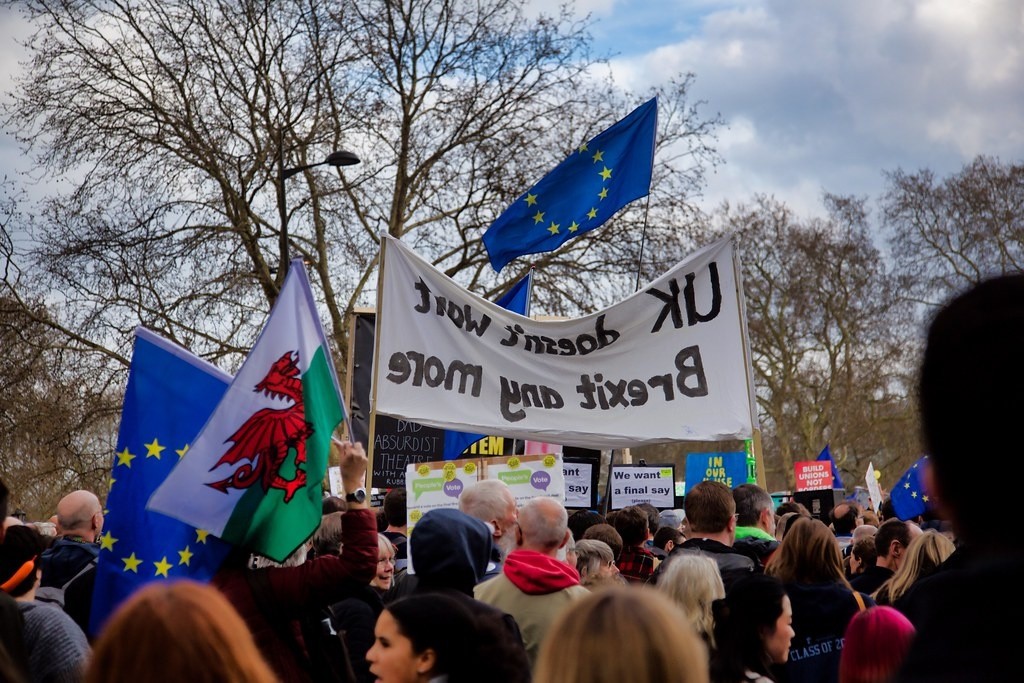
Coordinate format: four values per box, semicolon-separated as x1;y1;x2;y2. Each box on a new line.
38;557;101;608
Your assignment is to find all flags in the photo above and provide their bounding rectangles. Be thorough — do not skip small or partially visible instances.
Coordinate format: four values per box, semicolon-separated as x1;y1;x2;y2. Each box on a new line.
483;95;659;274
444;265;536;460
815;444;843;488
88;325;238;637
890;454;935;522
146;257;350;565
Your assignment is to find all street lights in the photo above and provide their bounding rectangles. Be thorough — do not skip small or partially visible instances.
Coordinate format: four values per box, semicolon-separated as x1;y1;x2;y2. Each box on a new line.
275;151;360;278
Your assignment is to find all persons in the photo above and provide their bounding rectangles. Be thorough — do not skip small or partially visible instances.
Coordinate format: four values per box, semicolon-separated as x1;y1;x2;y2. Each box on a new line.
886;268;1024;683
0;441;957;683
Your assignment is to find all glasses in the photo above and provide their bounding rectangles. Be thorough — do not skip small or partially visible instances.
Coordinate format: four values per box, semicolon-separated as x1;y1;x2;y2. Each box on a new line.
731;513;740;521
600;560;616;571
379;557;397;569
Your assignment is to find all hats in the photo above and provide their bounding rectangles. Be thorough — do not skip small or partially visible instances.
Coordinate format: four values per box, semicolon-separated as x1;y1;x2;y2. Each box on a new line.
657;509;686;532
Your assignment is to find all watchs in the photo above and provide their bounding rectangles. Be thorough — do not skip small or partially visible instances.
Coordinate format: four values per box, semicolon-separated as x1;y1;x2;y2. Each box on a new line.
346;488;367;504
